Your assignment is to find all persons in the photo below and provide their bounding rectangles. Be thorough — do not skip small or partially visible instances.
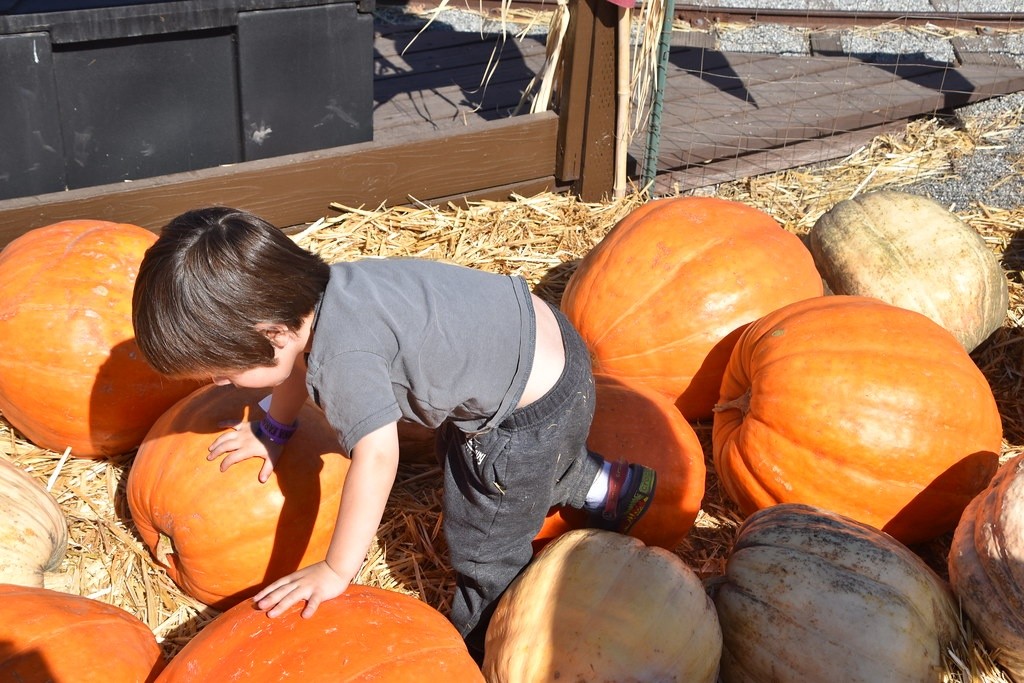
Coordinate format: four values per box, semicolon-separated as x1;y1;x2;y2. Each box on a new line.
132;205;657;669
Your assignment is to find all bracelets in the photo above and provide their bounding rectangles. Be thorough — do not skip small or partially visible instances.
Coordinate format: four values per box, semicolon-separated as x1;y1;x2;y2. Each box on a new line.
260;411;298;443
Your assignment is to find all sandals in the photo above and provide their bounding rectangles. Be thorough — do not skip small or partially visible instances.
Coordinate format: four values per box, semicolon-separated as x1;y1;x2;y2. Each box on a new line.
586;460;657;535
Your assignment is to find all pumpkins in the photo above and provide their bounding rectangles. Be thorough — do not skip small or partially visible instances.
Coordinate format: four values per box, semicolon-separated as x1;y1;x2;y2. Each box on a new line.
0;193;1024;683
